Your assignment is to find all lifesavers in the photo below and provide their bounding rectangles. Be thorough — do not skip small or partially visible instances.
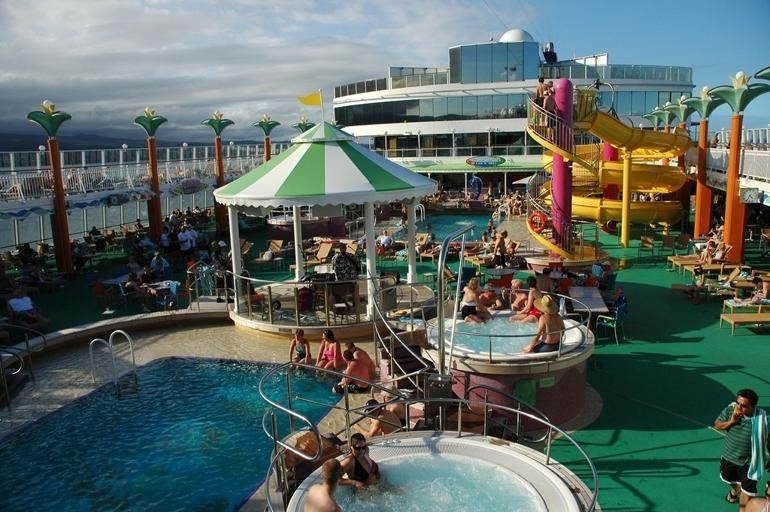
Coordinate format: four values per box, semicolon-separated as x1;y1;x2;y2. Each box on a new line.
527;212;548;233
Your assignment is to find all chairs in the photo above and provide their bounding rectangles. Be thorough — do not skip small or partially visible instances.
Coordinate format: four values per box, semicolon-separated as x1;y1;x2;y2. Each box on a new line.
639;227;770;335
0;210;254;342
258;228;630;348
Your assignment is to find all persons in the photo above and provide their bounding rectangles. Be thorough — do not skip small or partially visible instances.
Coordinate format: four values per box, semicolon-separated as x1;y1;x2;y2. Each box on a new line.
332;243;361;308
288;327;375;394
357;203;408;254
525;296;565;353
640;191;661;201
460;277;544;323
362;386;406;437
711;133;719;148
748;205;768;241
1;243;51;343
266;239;316;258
536;77;551;107
538;261;615;296
734;277;770;305
163;207;266;303
71;219;176;311
305;459;342;512
543;91;559;141
338;433;378;488
422;172;524;215
414;240;457;255
699;214;726;265
692;265;704;304
472;208;521;270
548;81;555;95
715;389;769;512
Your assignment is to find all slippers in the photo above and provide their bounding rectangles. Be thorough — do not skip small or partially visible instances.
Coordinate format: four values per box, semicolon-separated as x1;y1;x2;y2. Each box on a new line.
725;489;742;503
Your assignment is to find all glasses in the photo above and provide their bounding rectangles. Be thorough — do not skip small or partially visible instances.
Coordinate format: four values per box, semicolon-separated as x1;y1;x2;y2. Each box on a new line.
353;445;367;451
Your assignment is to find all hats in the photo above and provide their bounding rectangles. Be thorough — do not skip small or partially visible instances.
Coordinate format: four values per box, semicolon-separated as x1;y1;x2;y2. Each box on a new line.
533;294;559;315
218;241;228;247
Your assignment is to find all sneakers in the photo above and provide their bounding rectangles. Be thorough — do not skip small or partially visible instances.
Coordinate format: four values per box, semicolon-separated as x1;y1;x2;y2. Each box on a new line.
217;297;234;303
143;307;151;312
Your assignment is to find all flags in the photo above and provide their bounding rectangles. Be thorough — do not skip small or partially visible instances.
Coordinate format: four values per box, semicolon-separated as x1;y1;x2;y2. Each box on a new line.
297;91;321;105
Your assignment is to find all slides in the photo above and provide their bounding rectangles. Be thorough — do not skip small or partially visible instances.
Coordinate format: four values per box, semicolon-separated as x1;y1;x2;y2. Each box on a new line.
541;108;691;226
